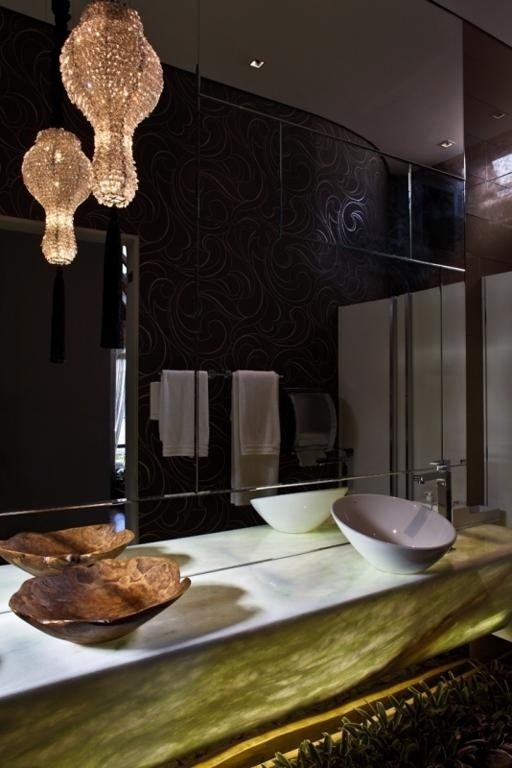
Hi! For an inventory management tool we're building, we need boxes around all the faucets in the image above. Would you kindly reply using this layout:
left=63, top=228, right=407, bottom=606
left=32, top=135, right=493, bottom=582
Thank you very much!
left=412, top=460, right=451, bottom=523
left=316, top=448, right=353, bottom=489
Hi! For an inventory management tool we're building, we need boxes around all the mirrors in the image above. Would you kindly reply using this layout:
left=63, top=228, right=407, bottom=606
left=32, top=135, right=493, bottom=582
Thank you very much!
left=0, top=0, right=467, bottom=565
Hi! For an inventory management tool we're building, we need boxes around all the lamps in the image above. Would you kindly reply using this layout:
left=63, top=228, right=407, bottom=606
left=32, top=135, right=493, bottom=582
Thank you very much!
left=59, top=0, right=165, bottom=349
left=20, top=1, right=94, bottom=363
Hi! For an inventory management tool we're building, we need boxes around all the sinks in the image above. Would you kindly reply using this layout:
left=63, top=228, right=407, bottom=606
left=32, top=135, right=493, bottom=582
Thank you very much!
left=250, top=486, right=349, bottom=533
left=332, top=493, right=455, bottom=575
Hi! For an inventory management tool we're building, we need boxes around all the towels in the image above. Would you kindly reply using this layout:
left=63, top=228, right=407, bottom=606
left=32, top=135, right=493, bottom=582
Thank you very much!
left=159, top=369, right=209, bottom=458
left=230, top=369, right=281, bottom=506
